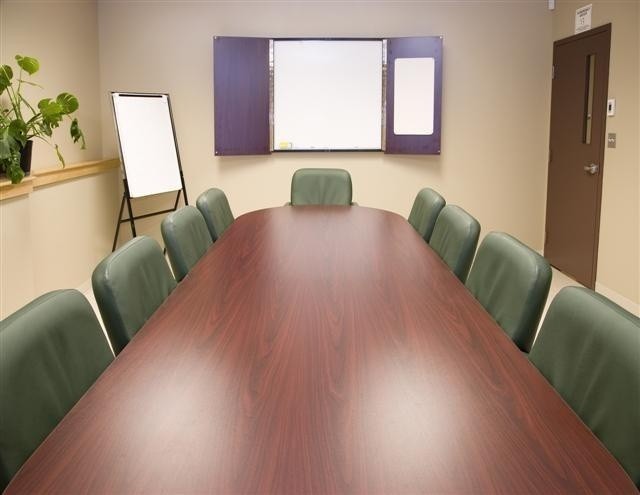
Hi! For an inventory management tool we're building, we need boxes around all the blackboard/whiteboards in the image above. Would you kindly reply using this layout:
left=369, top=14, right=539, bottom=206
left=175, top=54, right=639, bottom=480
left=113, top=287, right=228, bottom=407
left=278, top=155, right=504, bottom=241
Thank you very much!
left=109, top=91, right=184, bottom=198
left=269, top=39, right=387, bottom=150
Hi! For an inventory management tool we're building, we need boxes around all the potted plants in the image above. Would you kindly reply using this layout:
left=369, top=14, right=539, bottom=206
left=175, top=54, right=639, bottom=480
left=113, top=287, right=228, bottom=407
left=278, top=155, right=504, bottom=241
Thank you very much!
left=0, top=53, right=87, bottom=185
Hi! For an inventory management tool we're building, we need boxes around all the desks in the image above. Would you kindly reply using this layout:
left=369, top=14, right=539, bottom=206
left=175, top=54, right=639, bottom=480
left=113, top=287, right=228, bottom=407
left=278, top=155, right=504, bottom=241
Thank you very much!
left=0, top=204, right=640, bottom=495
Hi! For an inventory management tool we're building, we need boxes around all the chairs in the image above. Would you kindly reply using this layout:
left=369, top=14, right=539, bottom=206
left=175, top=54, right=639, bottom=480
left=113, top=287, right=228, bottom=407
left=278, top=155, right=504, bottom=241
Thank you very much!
left=281, top=167, right=361, bottom=208
left=528, top=283, right=640, bottom=491
left=0, top=285, right=117, bottom=494
left=159, top=205, right=215, bottom=283
left=407, top=187, right=447, bottom=243
left=462, top=228, right=554, bottom=352
left=90, top=234, right=177, bottom=357
left=195, top=186, right=237, bottom=243
left=428, top=204, right=481, bottom=286
